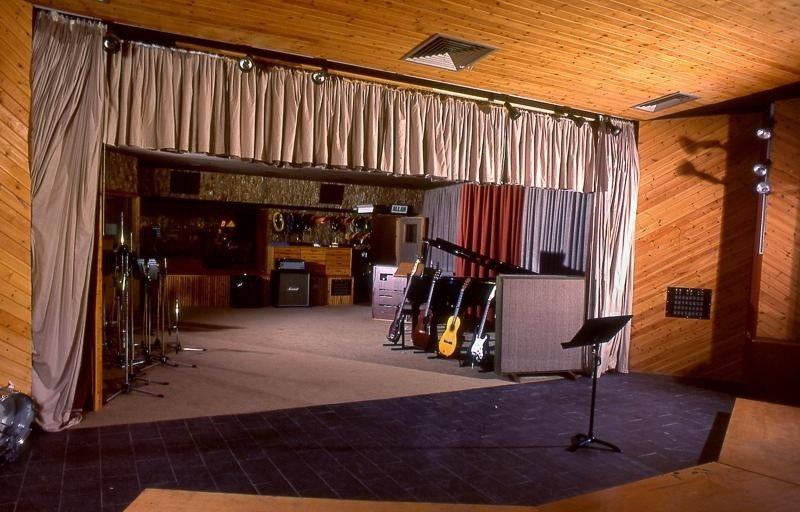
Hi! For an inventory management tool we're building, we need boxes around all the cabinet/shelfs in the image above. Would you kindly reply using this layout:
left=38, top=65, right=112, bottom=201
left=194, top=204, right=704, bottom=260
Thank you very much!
left=267, top=246, right=352, bottom=277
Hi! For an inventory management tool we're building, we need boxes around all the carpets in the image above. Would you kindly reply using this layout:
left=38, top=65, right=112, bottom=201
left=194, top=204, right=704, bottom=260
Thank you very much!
left=1, top=372, right=737, bottom=511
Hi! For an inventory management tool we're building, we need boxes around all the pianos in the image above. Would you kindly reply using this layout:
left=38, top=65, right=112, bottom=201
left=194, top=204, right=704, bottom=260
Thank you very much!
left=406, top=238, right=539, bottom=333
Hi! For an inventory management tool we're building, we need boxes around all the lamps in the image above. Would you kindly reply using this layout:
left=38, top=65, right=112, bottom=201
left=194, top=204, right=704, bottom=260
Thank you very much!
left=503, top=100, right=622, bottom=136
left=240, top=54, right=254, bottom=72
left=103, top=33, right=121, bottom=54
left=313, top=65, right=329, bottom=85
left=754, top=114, right=773, bottom=196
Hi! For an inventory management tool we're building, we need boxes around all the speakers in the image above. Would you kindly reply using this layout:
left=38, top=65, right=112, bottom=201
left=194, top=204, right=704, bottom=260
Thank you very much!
left=270, top=269, right=310, bottom=307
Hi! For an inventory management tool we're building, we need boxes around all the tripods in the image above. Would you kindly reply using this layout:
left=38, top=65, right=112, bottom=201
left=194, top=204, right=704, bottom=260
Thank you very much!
left=571, top=333, right=624, bottom=453
left=103, top=209, right=207, bottom=406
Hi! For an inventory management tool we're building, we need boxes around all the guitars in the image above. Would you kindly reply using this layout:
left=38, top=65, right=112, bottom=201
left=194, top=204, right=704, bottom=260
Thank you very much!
left=437, top=277, right=471, bottom=359
left=412, top=270, right=441, bottom=350
left=389, top=256, right=424, bottom=342
left=466, top=285, right=496, bottom=363
